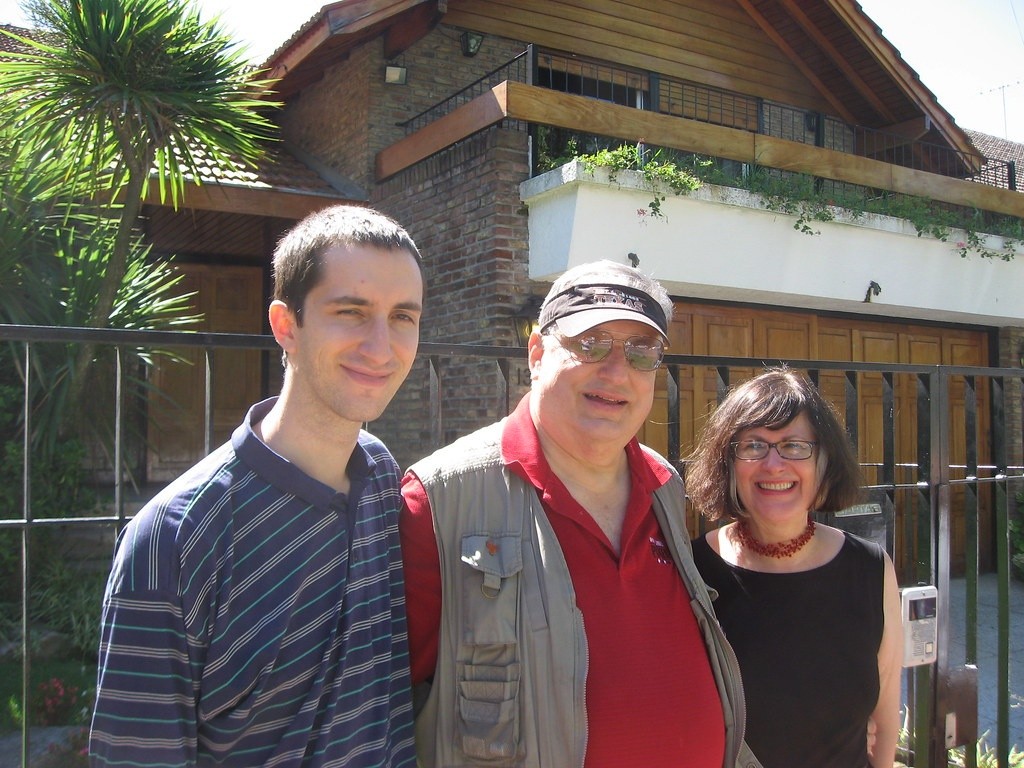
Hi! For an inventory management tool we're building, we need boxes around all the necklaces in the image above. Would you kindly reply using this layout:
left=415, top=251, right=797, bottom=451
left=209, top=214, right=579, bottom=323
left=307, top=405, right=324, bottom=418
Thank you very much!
left=736, top=517, right=816, bottom=560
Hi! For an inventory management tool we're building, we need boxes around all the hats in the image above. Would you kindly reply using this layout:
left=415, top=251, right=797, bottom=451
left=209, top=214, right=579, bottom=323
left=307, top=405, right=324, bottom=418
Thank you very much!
left=539, top=283, right=671, bottom=349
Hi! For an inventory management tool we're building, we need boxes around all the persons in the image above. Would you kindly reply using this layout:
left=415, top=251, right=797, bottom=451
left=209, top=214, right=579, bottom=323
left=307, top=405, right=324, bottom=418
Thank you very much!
left=688, top=371, right=903, bottom=768
left=88, top=207, right=425, bottom=768
left=402, top=261, right=879, bottom=768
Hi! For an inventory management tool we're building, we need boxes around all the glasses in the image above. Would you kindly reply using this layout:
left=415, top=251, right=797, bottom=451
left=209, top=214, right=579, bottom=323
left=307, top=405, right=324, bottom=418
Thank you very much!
left=731, top=439, right=820, bottom=461
left=540, top=329, right=665, bottom=372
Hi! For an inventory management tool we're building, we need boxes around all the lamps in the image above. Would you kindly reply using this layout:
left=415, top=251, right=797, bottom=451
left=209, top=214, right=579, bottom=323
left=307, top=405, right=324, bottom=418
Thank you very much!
left=806, top=109, right=818, bottom=131
left=460, top=32, right=484, bottom=56
left=384, top=53, right=407, bottom=85
